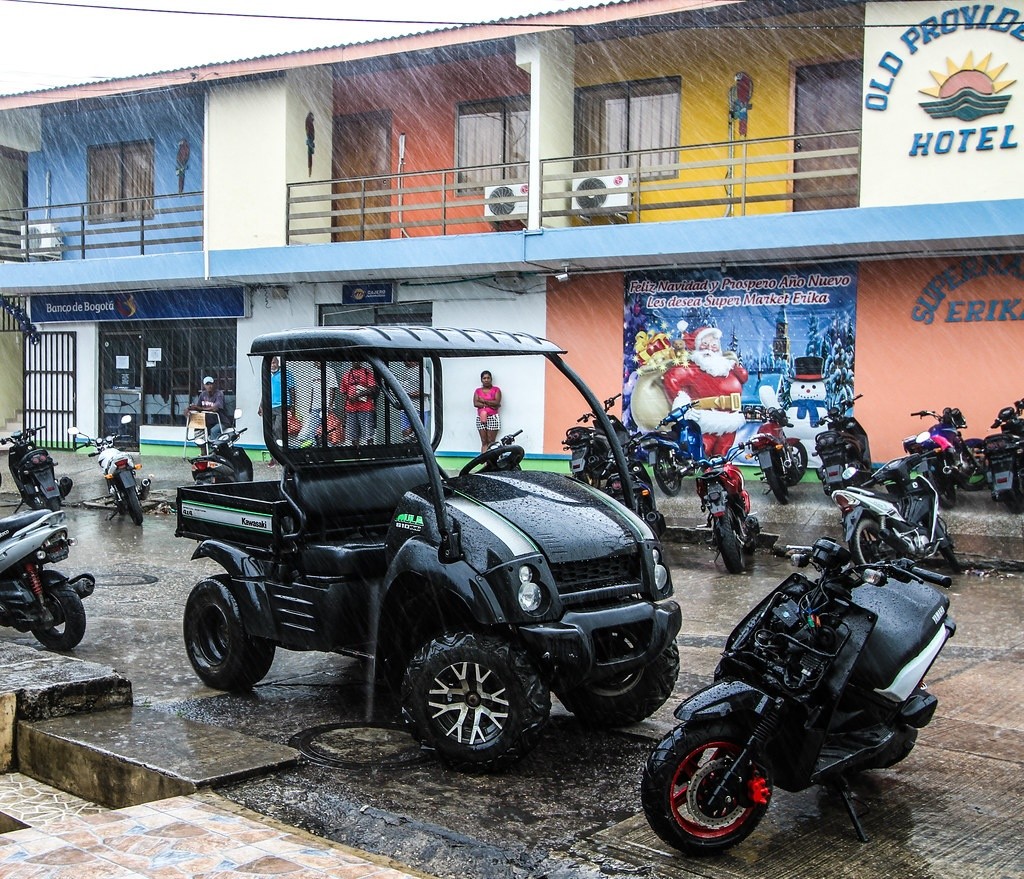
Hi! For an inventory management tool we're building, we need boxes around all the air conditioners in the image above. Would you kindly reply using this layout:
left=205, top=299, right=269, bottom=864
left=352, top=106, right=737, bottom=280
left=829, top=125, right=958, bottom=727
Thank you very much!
left=19, top=220, right=63, bottom=257
left=484, top=183, right=529, bottom=216
left=570, top=172, right=635, bottom=212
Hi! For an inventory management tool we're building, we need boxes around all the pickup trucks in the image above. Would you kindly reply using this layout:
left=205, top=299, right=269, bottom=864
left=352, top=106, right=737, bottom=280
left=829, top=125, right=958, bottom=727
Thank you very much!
left=174, top=323, right=684, bottom=774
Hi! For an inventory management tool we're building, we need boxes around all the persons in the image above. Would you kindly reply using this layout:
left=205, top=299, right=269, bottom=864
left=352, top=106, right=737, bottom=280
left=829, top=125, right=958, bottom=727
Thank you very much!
left=474, top=371, right=502, bottom=453
left=185, top=376, right=225, bottom=456
left=340, top=361, right=380, bottom=445
left=298, top=361, right=339, bottom=446
left=401, top=360, right=430, bottom=437
left=258, top=356, right=295, bottom=466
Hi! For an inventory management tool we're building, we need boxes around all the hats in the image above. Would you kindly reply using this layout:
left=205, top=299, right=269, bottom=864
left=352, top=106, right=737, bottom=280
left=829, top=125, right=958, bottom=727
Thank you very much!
left=203, top=376, right=214, bottom=385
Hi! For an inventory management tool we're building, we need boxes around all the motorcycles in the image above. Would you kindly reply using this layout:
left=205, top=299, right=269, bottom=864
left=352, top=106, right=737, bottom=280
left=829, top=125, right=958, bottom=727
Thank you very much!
left=0, top=509, right=96, bottom=652
left=188, top=408, right=254, bottom=485
left=562, top=393, right=707, bottom=535
left=67, top=415, right=152, bottom=526
left=830, top=431, right=961, bottom=576
left=0, top=423, right=73, bottom=519
left=679, top=439, right=761, bottom=575
left=905, top=398, right=1024, bottom=514
left=641, top=535, right=958, bottom=856
left=739, top=393, right=871, bottom=506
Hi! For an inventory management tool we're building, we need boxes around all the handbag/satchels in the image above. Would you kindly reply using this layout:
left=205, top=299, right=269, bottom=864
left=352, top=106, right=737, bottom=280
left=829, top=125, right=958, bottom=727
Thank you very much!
left=480, top=403, right=487, bottom=423
left=287, top=416, right=302, bottom=434
left=318, top=413, right=344, bottom=443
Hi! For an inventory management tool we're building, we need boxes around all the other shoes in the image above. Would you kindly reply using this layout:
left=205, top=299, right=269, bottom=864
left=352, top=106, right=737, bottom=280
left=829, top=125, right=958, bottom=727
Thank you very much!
left=267, top=459, right=275, bottom=468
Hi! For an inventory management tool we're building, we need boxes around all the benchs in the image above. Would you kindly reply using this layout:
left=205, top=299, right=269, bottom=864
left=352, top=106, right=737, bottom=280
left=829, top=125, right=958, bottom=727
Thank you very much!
left=294, top=458, right=429, bottom=574
